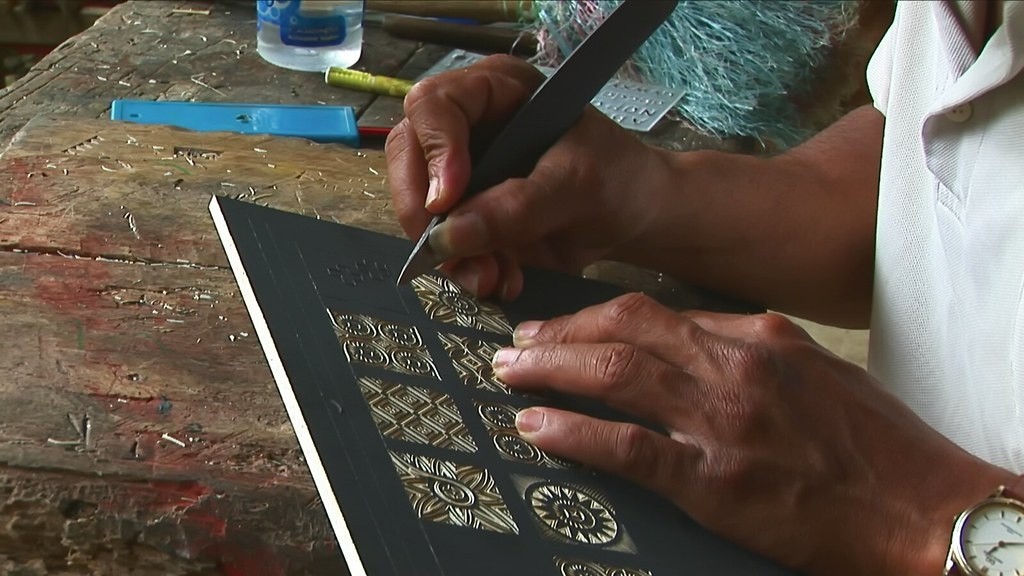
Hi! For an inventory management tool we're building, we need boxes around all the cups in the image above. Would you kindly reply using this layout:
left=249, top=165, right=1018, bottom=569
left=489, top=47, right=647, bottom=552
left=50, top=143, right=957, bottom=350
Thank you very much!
left=256, top=0, right=364, bottom=73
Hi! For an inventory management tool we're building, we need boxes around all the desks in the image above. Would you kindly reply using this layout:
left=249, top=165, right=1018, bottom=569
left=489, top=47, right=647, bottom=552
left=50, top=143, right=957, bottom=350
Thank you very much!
left=0, top=1, right=735, bottom=576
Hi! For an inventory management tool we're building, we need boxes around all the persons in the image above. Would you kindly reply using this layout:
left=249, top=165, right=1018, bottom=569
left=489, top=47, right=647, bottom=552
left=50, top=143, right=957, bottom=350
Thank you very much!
left=380, top=0, right=1023, bottom=574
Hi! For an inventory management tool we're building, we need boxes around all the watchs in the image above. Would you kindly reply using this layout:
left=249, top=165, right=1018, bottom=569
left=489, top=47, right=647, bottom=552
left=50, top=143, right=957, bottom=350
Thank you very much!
left=943, top=473, right=1024, bottom=576
